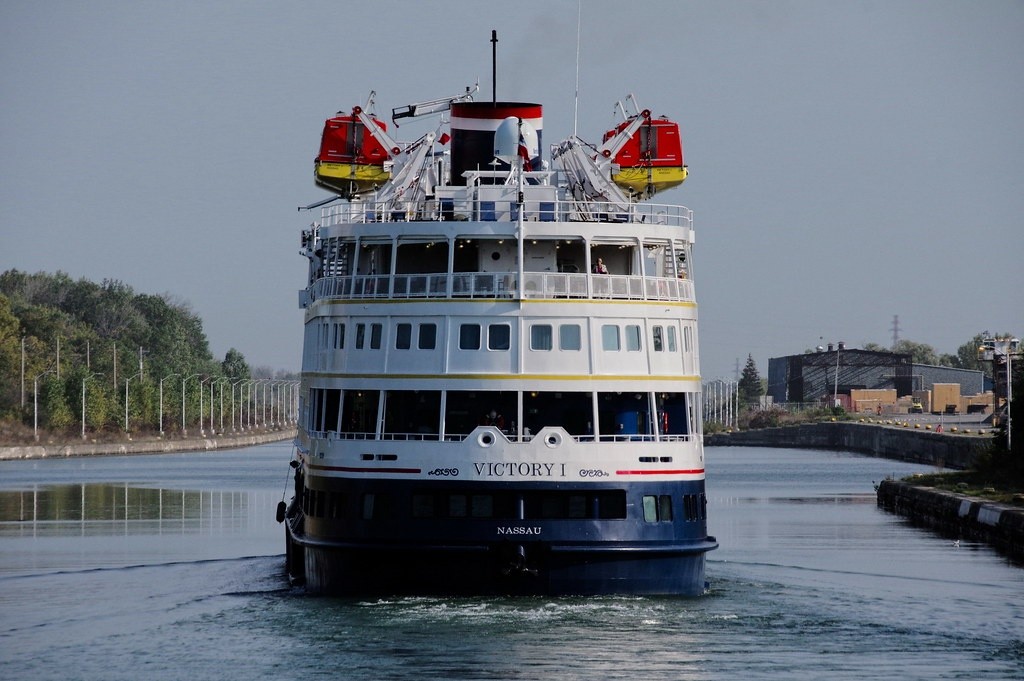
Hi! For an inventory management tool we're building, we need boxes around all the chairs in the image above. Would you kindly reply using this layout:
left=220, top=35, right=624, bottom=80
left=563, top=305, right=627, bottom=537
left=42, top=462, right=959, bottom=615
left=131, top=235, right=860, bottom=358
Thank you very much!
left=481, top=202, right=498, bottom=222
left=496, top=275, right=511, bottom=298
left=475, top=275, right=493, bottom=297
left=440, top=198, right=455, bottom=222
left=539, top=202, right=555, bottom=222
left=511, top=202, right=529, bottom=222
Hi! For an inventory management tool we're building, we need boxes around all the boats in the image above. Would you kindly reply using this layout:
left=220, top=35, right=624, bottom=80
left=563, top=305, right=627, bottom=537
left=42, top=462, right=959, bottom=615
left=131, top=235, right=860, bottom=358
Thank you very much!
left=283, top=26, right=722, bottom=601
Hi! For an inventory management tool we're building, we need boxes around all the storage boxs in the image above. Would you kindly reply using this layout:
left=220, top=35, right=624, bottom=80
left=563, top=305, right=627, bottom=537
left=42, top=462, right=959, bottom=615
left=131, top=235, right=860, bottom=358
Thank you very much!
left=392, top=212, right=407, bottom=221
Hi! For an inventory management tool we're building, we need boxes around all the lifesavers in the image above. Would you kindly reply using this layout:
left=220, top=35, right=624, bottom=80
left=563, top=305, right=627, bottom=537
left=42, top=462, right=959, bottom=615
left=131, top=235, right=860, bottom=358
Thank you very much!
left=484, top=412, right=505, bottom=430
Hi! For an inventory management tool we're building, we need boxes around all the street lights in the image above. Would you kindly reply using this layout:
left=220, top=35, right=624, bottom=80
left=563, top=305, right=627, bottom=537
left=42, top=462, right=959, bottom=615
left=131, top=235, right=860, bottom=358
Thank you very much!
left=160, top=375, right=181, bottom=433
left=125, top=371, right=141, bottom=433
left=34, top=370, right=57, bottom=440
left=182, top=373, right=203, bottom=433
left=702, top=380, right=739, bottom=431
left=82, top=371, right=104, bottom=434
left=978, top=344, right=1011, bottom=450
left=834, top=348, right=840, bottom=400
left=211, top=376, right=301, bottom=433
left=200, top=375, right=218, bottom=432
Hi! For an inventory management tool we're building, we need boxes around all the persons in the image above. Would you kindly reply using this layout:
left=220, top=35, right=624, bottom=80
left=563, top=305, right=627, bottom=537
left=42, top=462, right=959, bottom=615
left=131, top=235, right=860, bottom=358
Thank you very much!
left=591, top=258, right=608, bottom=299
left=485, top=410, right=506, bottom=430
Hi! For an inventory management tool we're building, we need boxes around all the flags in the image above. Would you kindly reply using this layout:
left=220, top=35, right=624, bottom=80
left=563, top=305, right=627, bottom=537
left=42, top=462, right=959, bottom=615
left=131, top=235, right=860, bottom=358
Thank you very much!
left=518, top=130, right=532, bottom=173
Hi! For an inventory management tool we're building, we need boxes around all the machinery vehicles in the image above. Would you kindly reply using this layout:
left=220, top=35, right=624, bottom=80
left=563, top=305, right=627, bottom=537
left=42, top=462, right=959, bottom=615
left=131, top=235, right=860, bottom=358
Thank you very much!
left=908, top=397, right=924, bottom=414
